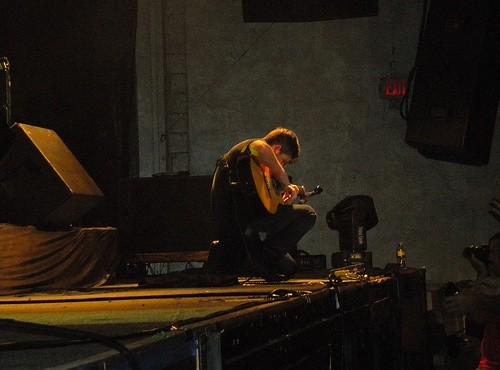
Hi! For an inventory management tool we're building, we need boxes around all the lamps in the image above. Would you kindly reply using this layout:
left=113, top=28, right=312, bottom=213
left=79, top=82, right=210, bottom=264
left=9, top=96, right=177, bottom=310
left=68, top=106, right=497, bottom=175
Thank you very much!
left=326, top=195, right=379, bottom=270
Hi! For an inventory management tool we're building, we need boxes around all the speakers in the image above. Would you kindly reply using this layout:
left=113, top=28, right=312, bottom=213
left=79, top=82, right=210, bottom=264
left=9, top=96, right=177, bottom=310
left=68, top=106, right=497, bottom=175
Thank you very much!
left=126, top=174, right=220, bottom=253
left=0, top=121, right=105, bottom=229
left=404, top=0, right=500, bottom=166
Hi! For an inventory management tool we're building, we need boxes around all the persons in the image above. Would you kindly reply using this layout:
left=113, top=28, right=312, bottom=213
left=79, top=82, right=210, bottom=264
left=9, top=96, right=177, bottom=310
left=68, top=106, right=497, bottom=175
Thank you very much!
left=210, top=126, right=317, bottom=274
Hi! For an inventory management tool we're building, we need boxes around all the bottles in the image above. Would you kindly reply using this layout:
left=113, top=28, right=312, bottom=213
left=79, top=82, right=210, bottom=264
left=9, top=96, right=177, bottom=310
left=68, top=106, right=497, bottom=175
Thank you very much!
left=397, top=242, right=406, bottom=270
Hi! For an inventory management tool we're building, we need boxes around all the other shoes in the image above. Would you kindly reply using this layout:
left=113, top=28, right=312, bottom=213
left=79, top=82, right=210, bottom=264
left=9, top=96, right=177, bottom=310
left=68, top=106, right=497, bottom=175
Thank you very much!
left=289, top=249, right=309, bottom=263
left=261, top=245, right=289, bottom=280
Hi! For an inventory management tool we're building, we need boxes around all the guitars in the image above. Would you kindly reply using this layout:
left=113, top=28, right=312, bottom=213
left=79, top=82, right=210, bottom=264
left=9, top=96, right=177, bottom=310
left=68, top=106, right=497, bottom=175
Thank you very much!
left=236, top=153, right=323, bottom=218
